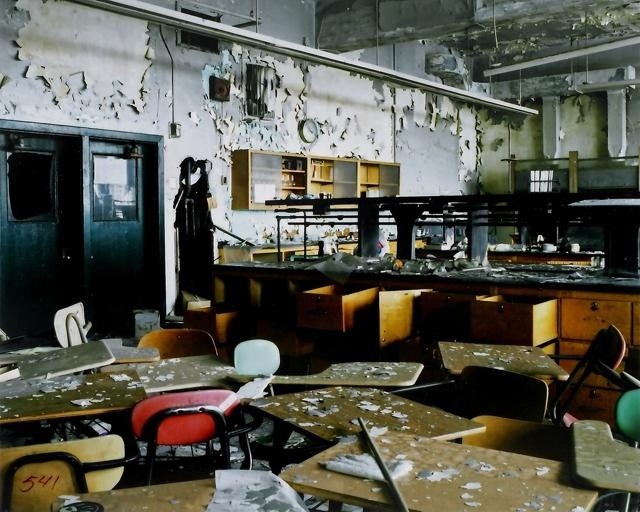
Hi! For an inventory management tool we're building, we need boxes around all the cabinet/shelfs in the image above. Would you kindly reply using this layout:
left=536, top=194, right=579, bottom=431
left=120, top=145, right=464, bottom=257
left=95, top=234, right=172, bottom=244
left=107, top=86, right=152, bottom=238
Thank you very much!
left=183, top=266, right=640, bottom=433
left=229, top=150, right=401, bottom=212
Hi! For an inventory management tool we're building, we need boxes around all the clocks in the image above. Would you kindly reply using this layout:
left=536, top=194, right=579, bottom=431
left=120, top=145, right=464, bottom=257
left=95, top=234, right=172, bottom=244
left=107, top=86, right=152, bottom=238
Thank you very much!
left=299, top=118, right=318, bottom=142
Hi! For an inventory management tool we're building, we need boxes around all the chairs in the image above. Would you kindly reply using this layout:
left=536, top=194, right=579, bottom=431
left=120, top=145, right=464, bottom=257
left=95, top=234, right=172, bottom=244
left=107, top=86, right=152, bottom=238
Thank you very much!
left=0, top=301, right=640, bottom=512
